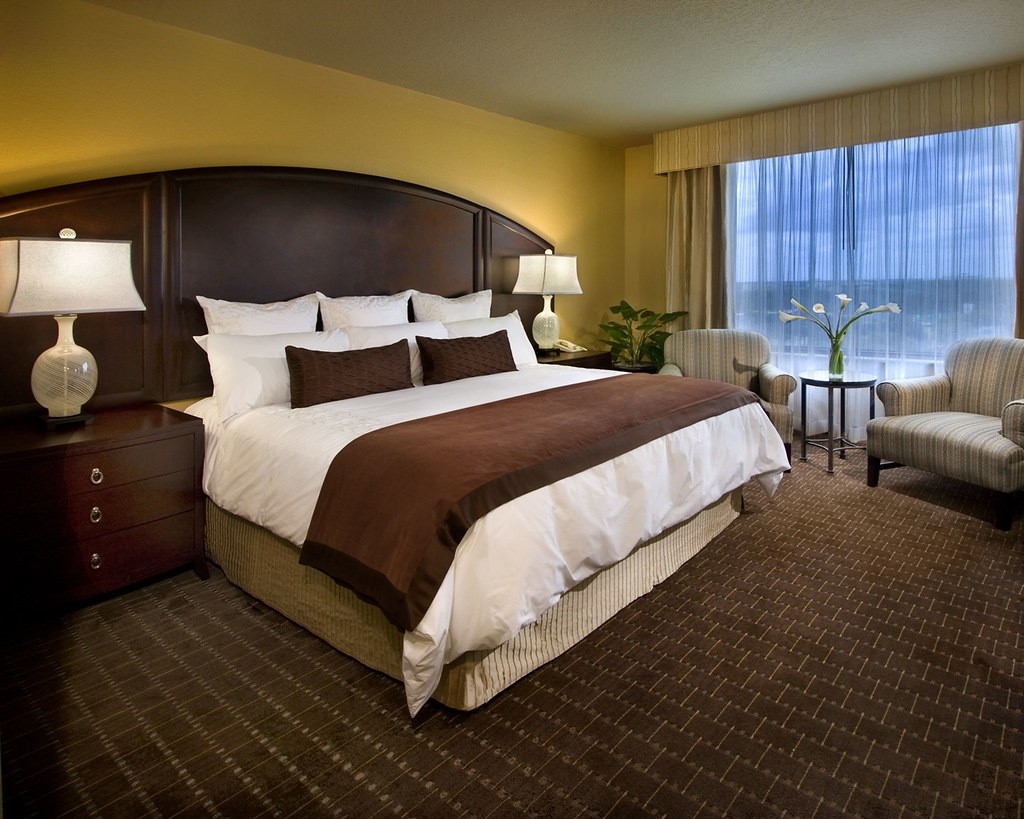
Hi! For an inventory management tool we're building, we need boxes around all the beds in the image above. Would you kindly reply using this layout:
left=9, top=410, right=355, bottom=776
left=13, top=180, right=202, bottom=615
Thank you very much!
left=184, top=363, right=792, bottom=719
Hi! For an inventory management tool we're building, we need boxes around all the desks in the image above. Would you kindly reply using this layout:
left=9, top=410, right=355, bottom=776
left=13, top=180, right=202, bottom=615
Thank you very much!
left=799, top=373, right=877, bottom=472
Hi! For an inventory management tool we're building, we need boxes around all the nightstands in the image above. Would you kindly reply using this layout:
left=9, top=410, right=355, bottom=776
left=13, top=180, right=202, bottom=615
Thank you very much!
left=537, top=349, right=610, bottom=369
left=0, top=402, right=211, bottom=625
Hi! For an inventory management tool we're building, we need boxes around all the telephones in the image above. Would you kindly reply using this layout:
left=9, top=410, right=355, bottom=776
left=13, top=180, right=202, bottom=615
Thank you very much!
left=553, top=338, right=582, bottom=353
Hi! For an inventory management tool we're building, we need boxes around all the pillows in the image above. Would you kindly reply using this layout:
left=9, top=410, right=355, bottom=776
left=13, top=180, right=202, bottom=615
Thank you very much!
left=344, top=319, right=450, bottom=389
left=192, top=329, right=350, bottom=426
left=286, top=339, right=416, bottom=409
left=409, top=289, right=492, bottom=324
left=196, top=294, right=320, bottom=333
left=415, top=330, right=519, bottom=386
left=316, top=289, right=414, bottom=331
left=445, top=309, right=538, bottom=367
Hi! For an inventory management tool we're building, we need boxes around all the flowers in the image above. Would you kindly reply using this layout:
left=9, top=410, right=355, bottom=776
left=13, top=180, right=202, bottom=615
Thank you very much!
left=778, top=294, right=902, bottom=375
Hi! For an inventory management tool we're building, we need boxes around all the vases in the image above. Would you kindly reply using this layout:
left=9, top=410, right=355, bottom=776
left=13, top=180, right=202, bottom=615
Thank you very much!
left=829, top=337, right=846, bottom=381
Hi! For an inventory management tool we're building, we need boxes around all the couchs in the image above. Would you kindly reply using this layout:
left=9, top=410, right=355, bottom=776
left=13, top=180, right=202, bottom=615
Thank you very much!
left=866, top=335, right=1024, bottom=532
left=657, top=328, right=797, bottom=473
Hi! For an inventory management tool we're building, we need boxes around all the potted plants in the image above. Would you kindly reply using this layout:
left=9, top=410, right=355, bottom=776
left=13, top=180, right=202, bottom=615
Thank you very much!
left=595, top=299, right=689, bottom=374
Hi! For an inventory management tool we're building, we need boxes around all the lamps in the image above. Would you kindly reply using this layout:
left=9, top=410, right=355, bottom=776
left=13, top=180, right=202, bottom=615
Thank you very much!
left=512, top=249, right=584, bottom=355
left=0, top=226, right=147, bottom=432
